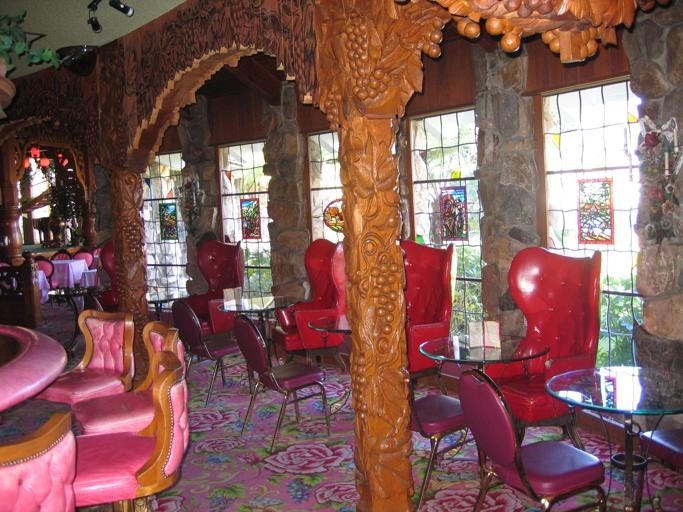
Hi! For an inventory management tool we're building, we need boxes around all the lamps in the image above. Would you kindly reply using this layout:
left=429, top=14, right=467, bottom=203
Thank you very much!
left=87, top=0, right=133, bottom=34
left=54, top=45, right=99, bottom=77
